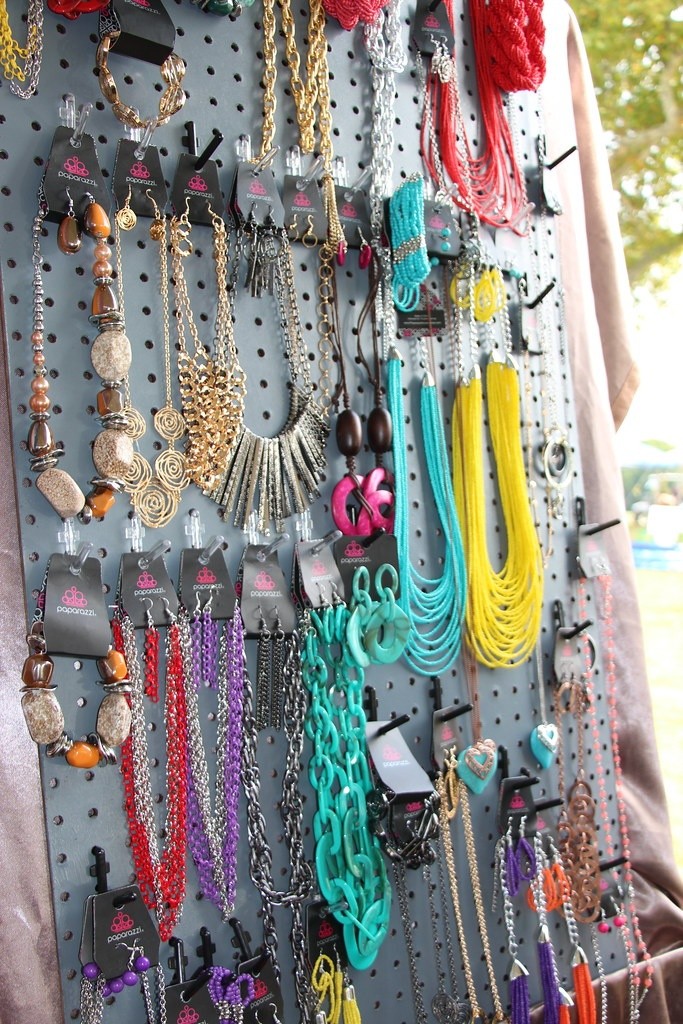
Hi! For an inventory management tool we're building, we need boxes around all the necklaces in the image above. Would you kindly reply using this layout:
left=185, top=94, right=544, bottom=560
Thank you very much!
left=281, top=0, right=320, bottom=154
left=172, top=201, right=247, bottom=489
left=0, top=0, right=44, bottom=99
left=174, top=0, right=624, bottom=675
left=262, top=0, right=276, bottom=164
left=30, top=190, right=132, bottom=523
left=71, top=520, right=650, bottom=1024
left=114, top=194, right=186, bottom=528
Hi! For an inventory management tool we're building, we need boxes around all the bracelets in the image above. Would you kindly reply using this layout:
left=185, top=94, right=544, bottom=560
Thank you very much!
left=92, top=29, right=186, bottom=130
left=21, top=622, right=130, bottom=767
